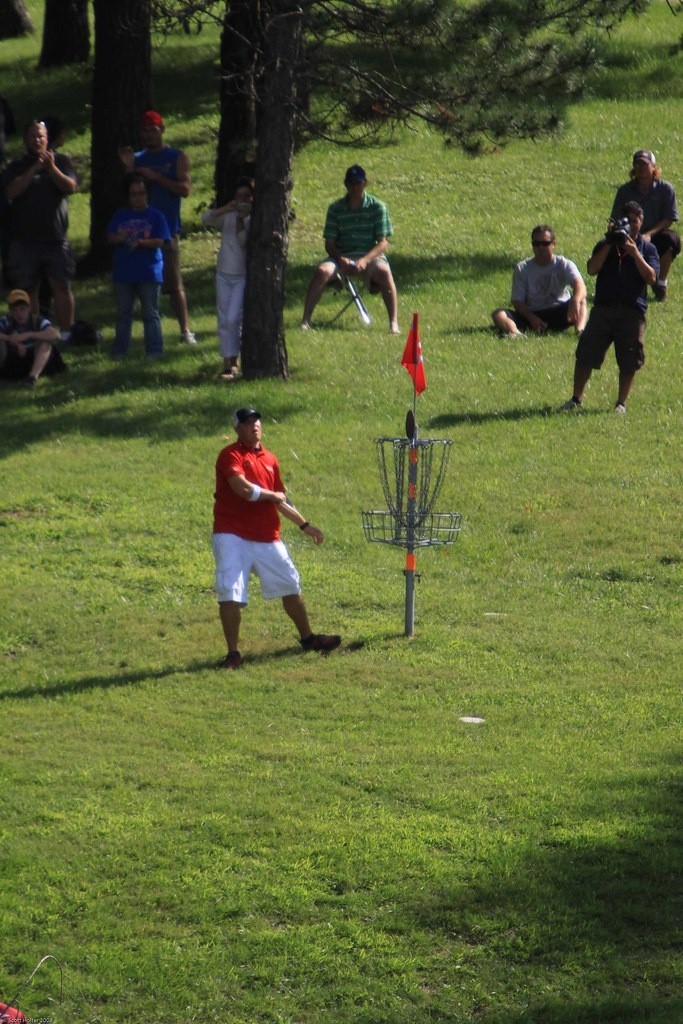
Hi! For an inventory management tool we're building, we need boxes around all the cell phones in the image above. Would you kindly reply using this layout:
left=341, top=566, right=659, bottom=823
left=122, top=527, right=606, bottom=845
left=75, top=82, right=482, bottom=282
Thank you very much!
left=605, top=218, right=631, bottom=245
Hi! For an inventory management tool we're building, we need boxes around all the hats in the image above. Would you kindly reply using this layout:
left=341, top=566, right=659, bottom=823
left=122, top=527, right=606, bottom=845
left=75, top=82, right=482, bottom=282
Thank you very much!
left=232, top=408, right=264, bottom=432
left=632, top=149, right=657, bottom=165
left=346, top=164, right=368, bottom=183
left=6, top=289, right=30, bottom=306
left=140, top=110, right=163, bottom=126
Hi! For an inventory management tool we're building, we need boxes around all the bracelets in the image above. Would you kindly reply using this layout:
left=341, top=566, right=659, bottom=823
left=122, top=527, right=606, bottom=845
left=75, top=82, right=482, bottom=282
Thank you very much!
left=248, top=483, right=260, bottom=502
left=299, top=522, right=309, bottom=530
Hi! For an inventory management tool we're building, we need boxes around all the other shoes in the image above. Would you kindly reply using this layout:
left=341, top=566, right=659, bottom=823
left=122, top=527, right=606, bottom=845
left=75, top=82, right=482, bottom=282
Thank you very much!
left=612, top=403, right=626, bottom=414
left=220, top=650, right=243, bottom=670
left=389, top=326, right=404, bottom=336
left=219, top=369, right=242, bottom=380
left=298, top=322, right=314, bottom=331
left=554, top=400, right=586, bottom=415
left=20, top=374, right=40, bottom=392
left=300, top=633, right=343, bottom=654
left=180, top=329, right=199, bottom=344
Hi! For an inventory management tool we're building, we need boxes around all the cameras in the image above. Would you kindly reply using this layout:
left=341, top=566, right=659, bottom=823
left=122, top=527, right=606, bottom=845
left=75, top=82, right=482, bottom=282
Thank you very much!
left=235, top=203, right=252, bottom=210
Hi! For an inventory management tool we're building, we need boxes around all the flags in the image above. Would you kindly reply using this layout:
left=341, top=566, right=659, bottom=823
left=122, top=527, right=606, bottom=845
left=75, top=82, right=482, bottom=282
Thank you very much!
left=401, top=313, right=426, bottom=395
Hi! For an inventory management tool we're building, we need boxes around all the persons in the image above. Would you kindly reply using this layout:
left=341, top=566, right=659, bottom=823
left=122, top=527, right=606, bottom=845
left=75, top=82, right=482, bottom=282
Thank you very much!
left=491, top=225, right=587, bottom=335
left=608, top=150, right=680, bottom=302
left=118, top=111, right=196, bottom=345
left=0, top=290, right=60, bottom=390
left=201, top=180, right=254, bottom=384
left=210, top=408, right=341, bottom=669
left=561, top=200, right=659, bottom=414
left=299, top=164, right=400, bottom=338
left=107, top=177, right=170, bottom=362
left=0, top=120, right=78, bottom=342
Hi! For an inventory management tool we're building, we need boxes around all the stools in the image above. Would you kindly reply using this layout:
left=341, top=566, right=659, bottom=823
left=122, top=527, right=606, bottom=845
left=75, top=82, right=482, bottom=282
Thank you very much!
left=326, top=274, right=372, bottom=326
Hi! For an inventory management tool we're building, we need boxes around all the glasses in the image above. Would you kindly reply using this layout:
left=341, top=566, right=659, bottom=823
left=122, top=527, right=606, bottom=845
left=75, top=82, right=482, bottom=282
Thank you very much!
left=531, top=240, right=551, bottom=247
